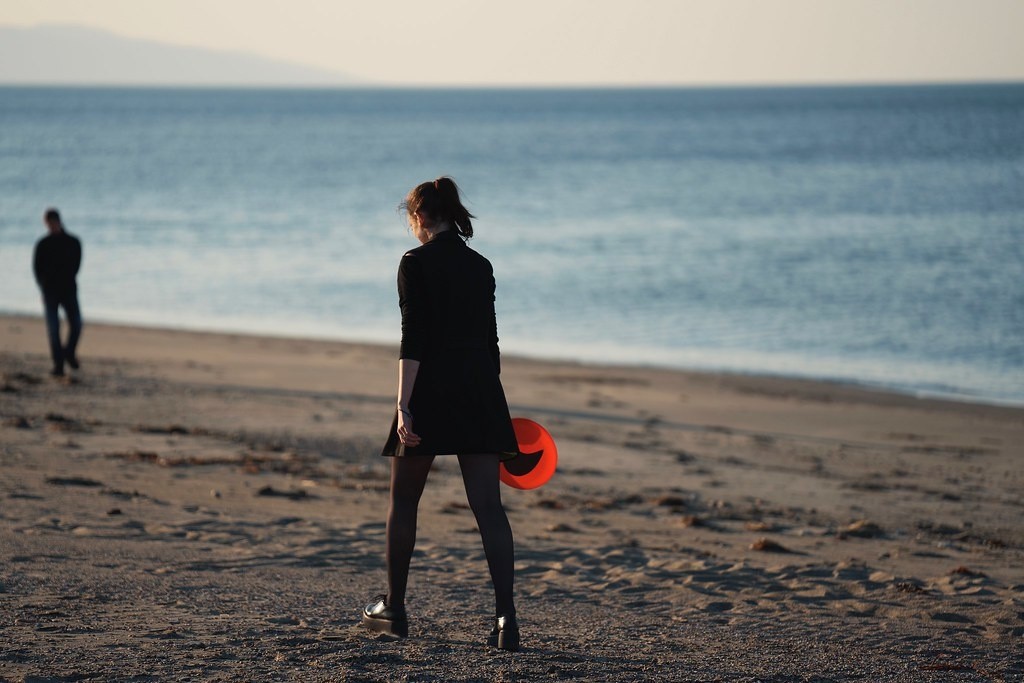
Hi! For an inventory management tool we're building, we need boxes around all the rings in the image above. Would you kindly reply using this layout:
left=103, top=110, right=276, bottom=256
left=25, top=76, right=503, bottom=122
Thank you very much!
left=400, top=435, right=405, bottom=438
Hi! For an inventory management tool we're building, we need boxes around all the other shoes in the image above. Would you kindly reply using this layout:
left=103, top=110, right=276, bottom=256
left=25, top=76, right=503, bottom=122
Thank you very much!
left=63, top=348, right=80, bottom=369
left=48, top=368, right=64, bottom=377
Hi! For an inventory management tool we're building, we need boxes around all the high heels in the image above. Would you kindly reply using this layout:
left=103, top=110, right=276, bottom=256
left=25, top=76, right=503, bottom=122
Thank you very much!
left=363, top=594, right=409, bottom=639
left=487, top=616, right=520, bottom=650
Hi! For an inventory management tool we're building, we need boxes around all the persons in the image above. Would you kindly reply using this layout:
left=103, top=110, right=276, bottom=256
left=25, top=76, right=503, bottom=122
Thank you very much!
left=33, top=211, right=82, bottom=377
left=363, top=176, right=522, bottom=651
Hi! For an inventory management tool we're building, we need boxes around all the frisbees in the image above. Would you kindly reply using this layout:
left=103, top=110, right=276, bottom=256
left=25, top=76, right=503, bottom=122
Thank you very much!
left=500, top=418, right=557, bottom=490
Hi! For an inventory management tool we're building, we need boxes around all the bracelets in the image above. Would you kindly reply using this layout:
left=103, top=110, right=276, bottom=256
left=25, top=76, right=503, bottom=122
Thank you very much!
left=396, top=408, right=414, bottom=421
